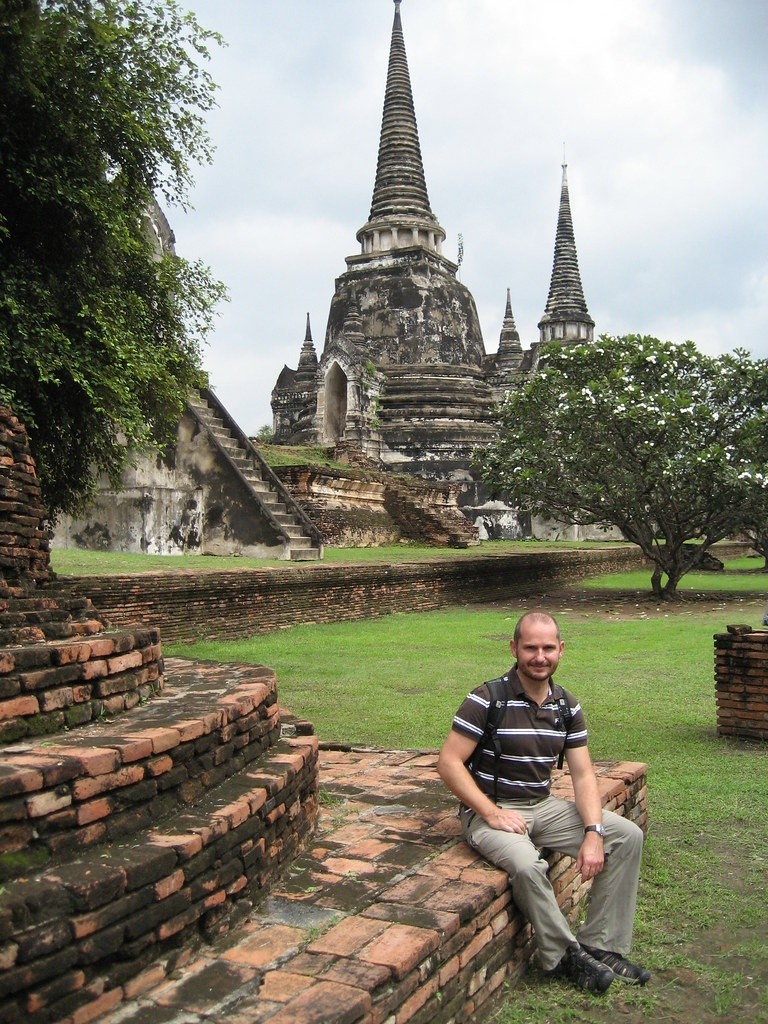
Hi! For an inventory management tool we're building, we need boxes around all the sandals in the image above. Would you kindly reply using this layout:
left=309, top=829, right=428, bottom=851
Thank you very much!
left=581, top=942, right=651, bottom=984
left=563, top=946, right=614, bottom=994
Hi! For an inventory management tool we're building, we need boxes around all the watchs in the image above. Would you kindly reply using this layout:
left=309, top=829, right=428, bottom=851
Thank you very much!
left=585, top=824, right=606, bottom=838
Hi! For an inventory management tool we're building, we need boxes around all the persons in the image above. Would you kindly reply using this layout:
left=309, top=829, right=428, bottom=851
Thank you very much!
left=437, top=612, right=651, bottom=996
